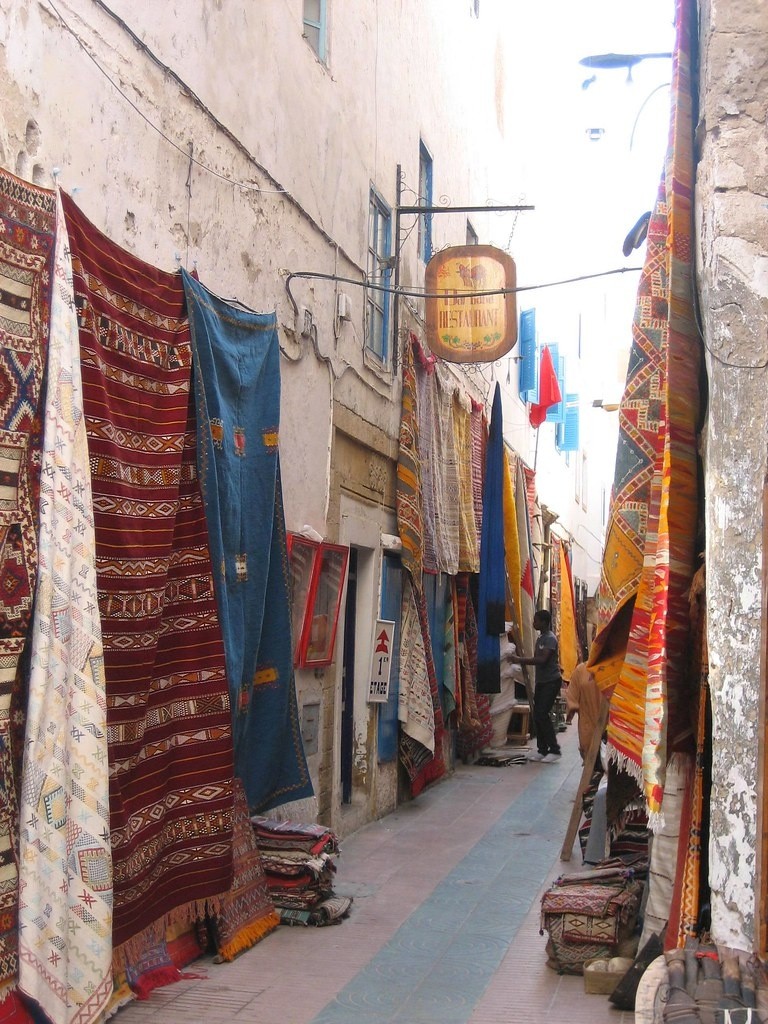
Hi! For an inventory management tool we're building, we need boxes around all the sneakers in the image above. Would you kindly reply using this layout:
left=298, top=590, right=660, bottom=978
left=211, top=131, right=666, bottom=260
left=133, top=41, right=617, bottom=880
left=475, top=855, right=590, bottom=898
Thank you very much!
left=528, top=753, right=545, bottom=761
left=540, top=752, right=561, bottom=762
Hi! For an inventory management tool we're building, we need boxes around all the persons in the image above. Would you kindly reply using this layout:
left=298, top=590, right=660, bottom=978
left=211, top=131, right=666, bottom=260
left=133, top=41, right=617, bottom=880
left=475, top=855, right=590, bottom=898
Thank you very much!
left=506, top=610, right=562, bottom=763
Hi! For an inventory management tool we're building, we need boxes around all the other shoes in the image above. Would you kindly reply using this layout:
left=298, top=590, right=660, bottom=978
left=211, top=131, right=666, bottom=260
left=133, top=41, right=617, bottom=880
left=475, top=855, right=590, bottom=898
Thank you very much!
left=564, top=721, right=572, bottom=725
